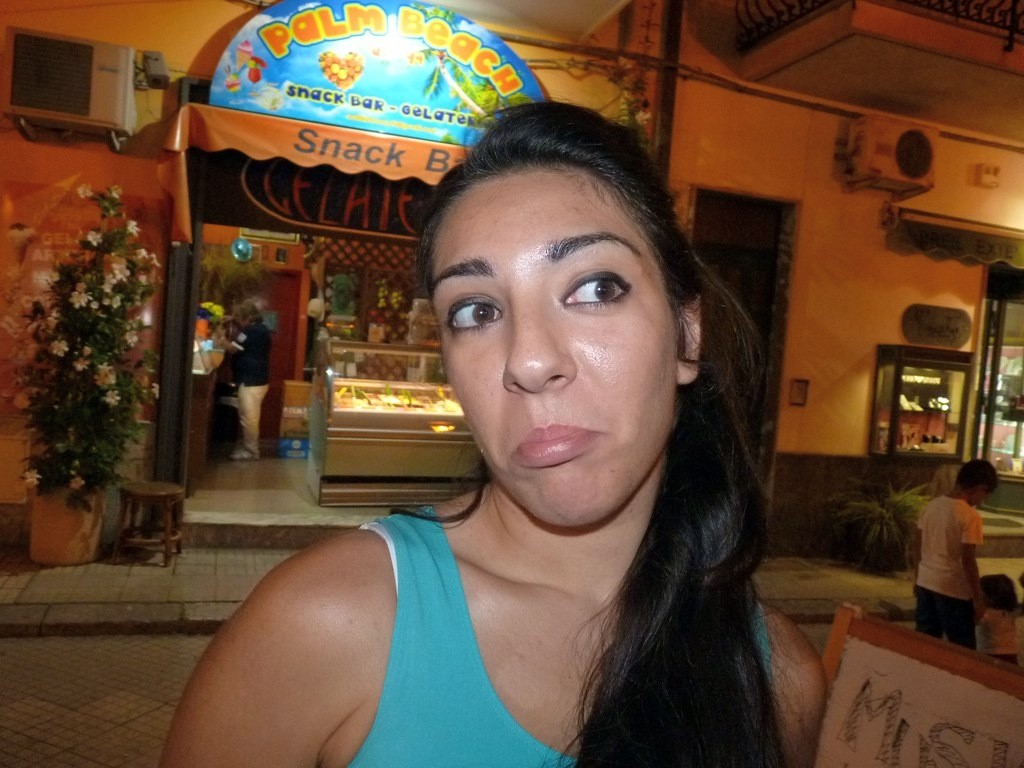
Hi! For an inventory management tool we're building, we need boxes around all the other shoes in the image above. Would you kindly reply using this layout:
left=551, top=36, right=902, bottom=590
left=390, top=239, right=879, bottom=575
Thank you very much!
left=229, top=448, right=260, bottom=460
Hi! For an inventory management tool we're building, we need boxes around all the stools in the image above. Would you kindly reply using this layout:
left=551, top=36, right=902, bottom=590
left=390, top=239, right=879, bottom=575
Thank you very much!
left=113, top=480, right=186, bottom=569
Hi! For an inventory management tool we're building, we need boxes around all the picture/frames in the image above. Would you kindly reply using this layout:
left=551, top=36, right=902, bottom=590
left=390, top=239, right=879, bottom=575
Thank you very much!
left=250, top=243, right=262, bottom=263
left=239, top=226, right=300, bottom=245
left=274, top=247, right=289, bottom=264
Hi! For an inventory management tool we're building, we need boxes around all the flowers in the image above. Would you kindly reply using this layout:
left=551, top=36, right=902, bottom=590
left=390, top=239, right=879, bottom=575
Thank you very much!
left=7, top=184, right=162, bottom=512
left=369, top=277, right=402, bottom=327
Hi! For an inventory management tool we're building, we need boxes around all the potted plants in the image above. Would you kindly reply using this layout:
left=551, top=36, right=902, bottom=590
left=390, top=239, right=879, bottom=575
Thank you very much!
left=325, top=274, right=356, bottom=340
left=839, top=481, right=936, bottom=572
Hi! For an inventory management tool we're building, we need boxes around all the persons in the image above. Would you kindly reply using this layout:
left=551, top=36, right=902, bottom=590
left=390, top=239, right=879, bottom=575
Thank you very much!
left=156, top=98, right=829, bottom=768
left=912, top=457, right=1022, bottom=667
left=217, top=298, right=273, bottom=461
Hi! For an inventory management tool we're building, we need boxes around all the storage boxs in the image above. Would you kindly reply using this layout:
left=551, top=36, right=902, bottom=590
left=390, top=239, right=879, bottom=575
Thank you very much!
left=280, top=416, right=311, bottom=439
left=280, top=439, right=309, bottom=459
left=280, top=379, right=312, bottom=418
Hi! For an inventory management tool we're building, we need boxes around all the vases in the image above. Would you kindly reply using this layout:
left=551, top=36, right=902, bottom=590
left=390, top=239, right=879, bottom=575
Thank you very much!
left=28, top=484, right=107, bottom=566
left=369, top=323, right=388, bottom=343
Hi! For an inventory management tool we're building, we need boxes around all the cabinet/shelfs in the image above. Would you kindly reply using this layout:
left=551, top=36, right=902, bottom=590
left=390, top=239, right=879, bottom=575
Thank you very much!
left=308, top=338, right=483, bottom=508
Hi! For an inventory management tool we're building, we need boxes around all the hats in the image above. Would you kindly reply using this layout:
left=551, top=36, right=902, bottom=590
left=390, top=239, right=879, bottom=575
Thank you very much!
left=307, top=289, right=324, bottom=322
left=232, top=299, right=262, bottom=321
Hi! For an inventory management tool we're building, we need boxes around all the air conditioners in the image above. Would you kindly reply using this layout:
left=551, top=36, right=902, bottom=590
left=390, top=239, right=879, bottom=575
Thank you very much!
left=847, top=113, right=939, bottom=192
